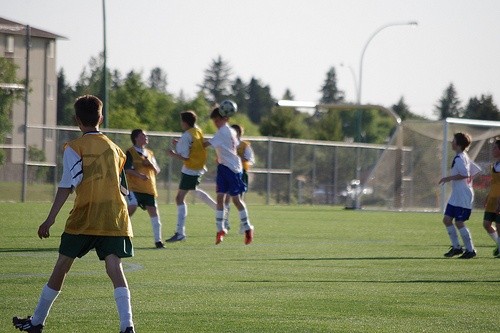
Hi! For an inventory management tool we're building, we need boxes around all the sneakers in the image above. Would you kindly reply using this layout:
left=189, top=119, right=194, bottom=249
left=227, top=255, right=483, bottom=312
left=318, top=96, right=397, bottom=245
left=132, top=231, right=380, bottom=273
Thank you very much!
left=120, top=326, right=135, bottom=333
left=493, top=248, right=500, bottom=257
left=459, top=250, right=476, bottom=258
left=12, top=315, right=45, bottom=333
left=444, top=245, right=463, bottom=257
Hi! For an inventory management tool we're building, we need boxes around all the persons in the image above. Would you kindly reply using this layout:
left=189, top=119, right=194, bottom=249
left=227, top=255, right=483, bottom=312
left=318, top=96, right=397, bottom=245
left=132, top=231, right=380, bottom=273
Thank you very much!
left=165, top=111, right=216, bottom=242
left=124, top=129, right=166, bottom=248
left=225, top=124, right=255, bottom=230
left=204, top=108, right=254, bottom=245
left=13, top=95, right=135, bottom=333
left=484, top=139, right=500, bottom=258
left=439, top=132, right=482, bottom=259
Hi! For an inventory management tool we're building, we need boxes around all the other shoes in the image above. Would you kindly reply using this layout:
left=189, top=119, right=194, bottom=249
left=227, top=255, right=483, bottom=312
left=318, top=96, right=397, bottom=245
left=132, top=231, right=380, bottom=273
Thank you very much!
left=216, top=229, right=226, bottom=245
left=244, top=228, right=253, bottom=244
left=166, top=232, right=185, bottom=241
left=155, top=241, right=166, bottom=248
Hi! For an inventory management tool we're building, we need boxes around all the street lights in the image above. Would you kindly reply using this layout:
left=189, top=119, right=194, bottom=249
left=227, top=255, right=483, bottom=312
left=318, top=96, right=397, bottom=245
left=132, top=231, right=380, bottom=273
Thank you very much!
left=355, top=20, right=419, bottom=179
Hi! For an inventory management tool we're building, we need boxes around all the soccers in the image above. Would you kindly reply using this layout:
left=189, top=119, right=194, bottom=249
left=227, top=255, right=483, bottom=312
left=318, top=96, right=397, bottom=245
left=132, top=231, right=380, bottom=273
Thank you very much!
left=218, top=99, right=239, bottom=119
